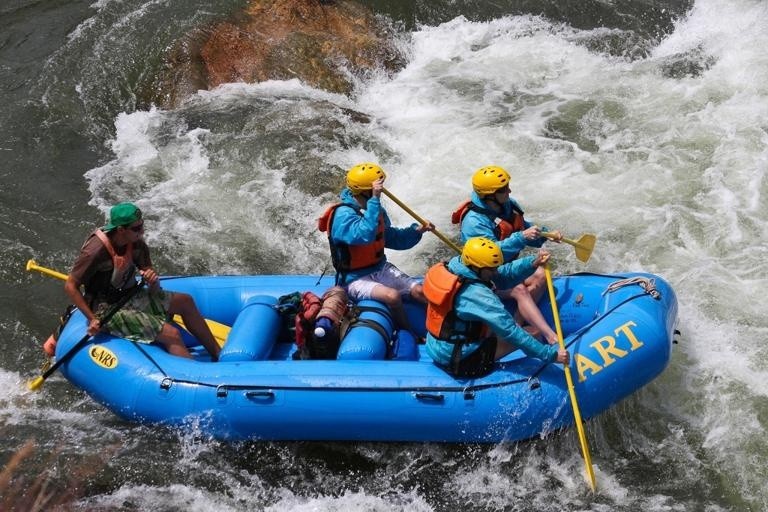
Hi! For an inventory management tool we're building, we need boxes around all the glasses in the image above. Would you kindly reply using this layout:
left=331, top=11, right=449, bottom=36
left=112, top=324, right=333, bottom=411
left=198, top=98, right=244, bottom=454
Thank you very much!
left=125, top=218, right=144, bottom=233
left=495, top=183, right=509, bottom=193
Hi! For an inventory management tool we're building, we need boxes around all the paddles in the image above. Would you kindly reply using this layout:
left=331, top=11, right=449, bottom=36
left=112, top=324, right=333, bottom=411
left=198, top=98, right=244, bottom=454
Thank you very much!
left=25, top=259, right=231, bottom=350
left=542, top=232, right=596, bottom=263
left=542, top=256, right=597, bottom=493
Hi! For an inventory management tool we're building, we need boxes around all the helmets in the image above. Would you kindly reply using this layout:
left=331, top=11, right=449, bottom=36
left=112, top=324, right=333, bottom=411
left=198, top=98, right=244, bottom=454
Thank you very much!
left=462, top=237, right=504, bottom=268
left=344, top=162, right=386, bottom=196
left=472, top=165, right=511, bottom=194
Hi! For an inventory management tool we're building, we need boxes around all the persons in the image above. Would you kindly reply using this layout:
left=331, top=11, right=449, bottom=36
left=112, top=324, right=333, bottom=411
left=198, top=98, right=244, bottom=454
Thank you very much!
left=326, top=164, right=437, bottom=346
left=63, top=202, right=222, bottom=362
left=459, top=166, right=563, bottom=345
left=426, top=237, right=571, bottom=377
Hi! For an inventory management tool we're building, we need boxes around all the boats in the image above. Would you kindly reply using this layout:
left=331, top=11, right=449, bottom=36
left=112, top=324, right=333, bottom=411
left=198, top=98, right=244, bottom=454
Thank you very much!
left=55, top=271, right=676, bottom=446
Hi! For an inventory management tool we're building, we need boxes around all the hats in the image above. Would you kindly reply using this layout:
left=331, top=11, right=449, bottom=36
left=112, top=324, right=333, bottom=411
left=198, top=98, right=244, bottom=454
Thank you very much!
left=98, top=202, right=141, bottom=231
